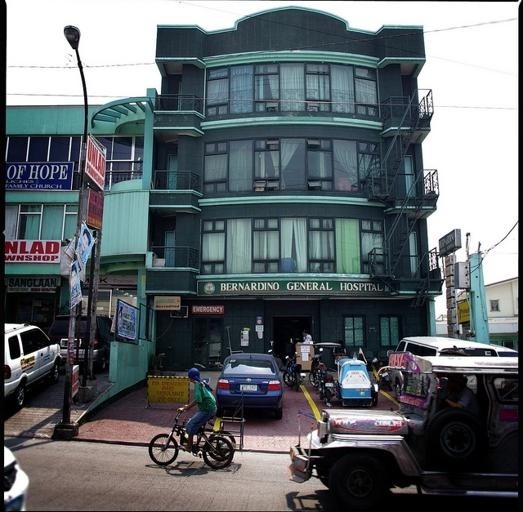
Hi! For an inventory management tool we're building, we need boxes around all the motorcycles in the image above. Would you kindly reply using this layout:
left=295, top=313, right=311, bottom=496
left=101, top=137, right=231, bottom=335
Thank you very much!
left=284, top=353, right=302, bottom=391
left=307, top=349, right=327, bottom=387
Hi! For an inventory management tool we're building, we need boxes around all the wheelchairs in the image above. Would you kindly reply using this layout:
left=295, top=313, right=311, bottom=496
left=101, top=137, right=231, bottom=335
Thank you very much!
left=316, top=375, right=341, bottom=407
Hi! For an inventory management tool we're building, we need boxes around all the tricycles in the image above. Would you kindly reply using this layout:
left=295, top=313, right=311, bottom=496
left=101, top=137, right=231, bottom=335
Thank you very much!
left=149, top=407, right=238, bottom=470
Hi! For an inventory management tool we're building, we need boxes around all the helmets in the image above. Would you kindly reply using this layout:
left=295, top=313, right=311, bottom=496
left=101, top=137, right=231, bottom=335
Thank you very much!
left=188, top=368, right=200, bottom=378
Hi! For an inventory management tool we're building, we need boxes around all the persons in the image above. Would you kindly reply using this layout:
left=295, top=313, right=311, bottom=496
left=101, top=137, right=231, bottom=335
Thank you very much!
left=71, top=264, right=79, bottom=294
left=446, top=376, right=474, bottom=409
left=177, top=368, right=218, bottom=452
left=302, top=329, right=312, bottom=343
left=80, top=228, right=91, bottom=258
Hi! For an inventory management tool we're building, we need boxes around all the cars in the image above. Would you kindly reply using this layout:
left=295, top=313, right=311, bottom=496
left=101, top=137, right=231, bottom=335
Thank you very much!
left=338, top=358, right=379, bottom=407
left=215, top=352, right=283, bottom=419
left=290, top=352, right=522, bottom=512
left=4, top=322, right=63, bottom=406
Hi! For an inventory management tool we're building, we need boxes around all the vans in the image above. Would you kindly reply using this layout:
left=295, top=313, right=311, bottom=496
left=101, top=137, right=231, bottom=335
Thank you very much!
left=387, top=336, right=499, bottom=358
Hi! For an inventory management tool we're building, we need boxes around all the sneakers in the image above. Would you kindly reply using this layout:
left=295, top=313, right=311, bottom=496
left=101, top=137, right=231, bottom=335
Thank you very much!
left=180, top=445, right=192, bottom=452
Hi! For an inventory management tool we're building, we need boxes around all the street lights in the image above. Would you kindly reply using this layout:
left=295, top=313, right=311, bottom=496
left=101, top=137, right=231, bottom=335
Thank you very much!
left=54, top=26, right=89, bottom=437
